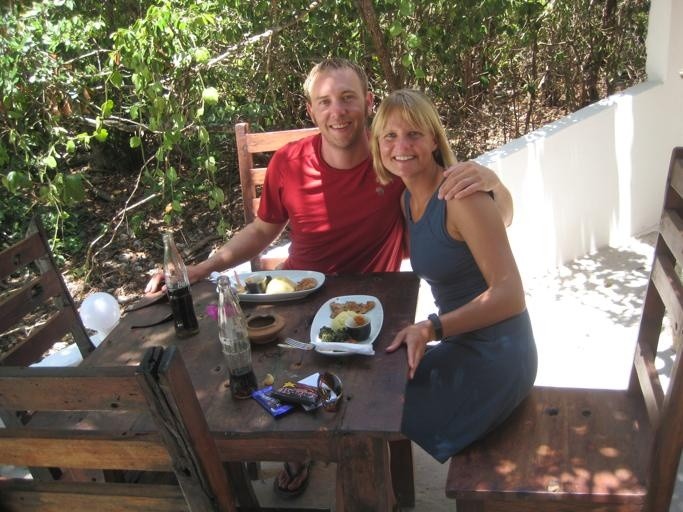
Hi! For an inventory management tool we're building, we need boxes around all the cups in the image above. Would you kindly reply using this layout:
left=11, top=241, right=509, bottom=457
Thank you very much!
left=317, top=372, right=344, bottom=411
left=123, top=292, right=175, bottom=329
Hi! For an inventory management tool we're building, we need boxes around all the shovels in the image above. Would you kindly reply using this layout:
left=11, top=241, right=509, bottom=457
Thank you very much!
left=295, top=278, right=318, bottom=291
left=329, top=301, right=375, bottom=319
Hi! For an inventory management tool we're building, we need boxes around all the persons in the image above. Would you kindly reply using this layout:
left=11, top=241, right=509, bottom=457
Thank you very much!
left=142, top=58, right=514, bottom=500
left=369, top=89, right=537, bottom=512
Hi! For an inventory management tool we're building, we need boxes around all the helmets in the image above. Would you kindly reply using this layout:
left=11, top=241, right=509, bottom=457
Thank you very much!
left=216, top=270, right=326, bottom=304
left=311, top=294, right=384, bottom=356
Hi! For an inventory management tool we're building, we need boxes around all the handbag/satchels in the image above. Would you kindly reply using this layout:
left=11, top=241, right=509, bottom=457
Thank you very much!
left=428, top=313, right=443, bottom=341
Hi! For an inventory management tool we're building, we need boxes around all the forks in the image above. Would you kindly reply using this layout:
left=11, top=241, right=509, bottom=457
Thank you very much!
left=285, top=337, right=315, bottom=351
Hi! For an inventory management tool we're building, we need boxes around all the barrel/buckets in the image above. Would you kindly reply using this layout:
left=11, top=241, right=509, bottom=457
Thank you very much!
left=274, top=462, right=311, bottom=497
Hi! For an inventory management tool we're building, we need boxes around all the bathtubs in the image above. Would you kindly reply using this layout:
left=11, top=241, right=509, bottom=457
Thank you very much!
left=318, top=325, right=348, bottom=342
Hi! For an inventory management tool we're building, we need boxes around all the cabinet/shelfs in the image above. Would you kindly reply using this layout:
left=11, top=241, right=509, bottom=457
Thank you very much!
left=217, top=275, right=258, bottom=401
left=160, top=230, right=200, bottom=338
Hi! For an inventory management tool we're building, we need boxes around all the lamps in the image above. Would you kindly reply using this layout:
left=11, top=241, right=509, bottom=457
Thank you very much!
left=446, top=148, right=683, bottom=512
left=234, top=123, right=321, bottom=272
left=0, top=214, right=96, bottom=481
left=0, top=345, right=237, bottom=512
